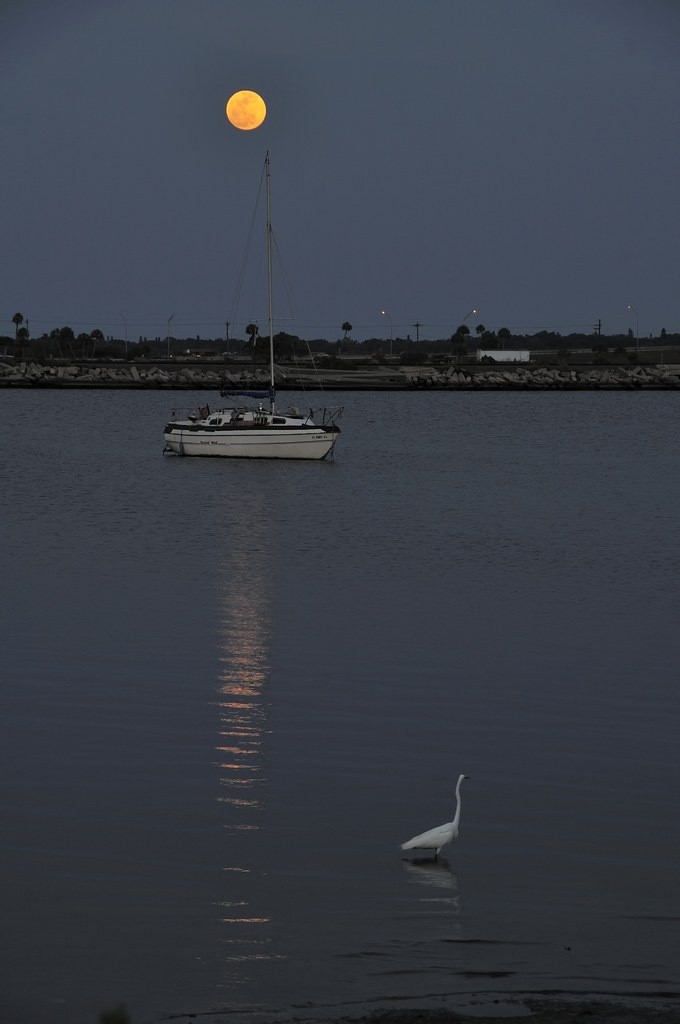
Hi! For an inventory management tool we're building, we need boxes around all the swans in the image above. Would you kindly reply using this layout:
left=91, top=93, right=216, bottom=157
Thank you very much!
left=398, top=773, right=470, bottom=860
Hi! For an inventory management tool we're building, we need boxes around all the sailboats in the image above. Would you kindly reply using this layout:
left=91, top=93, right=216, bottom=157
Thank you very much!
left=163, top=147, right=342, bottom=458
